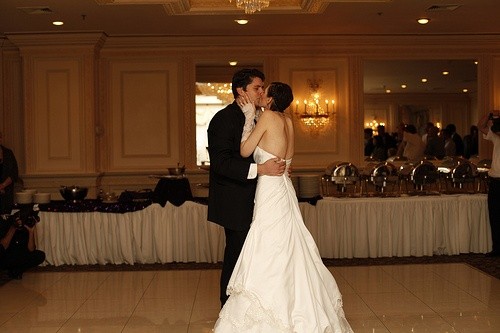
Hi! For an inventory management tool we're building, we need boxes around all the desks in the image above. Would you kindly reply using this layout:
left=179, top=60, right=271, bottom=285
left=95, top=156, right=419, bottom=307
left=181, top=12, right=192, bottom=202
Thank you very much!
left=34, top=194, right=493, bottom=267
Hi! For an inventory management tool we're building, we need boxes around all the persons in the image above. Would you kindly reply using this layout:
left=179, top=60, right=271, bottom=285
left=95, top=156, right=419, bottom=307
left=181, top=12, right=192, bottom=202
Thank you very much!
left=0, top=144, right=46, bottom=281
left=206, top=67, right=295, bottom=309
left=212, top=82, right=356, bottom=333
left=483, top=109, right=500, bottom=257
left=364, top=122, right=480, bottom=161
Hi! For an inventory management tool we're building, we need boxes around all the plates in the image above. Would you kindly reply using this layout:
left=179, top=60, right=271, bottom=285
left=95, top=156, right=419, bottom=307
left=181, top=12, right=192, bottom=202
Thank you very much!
left=22, top=189, right=38, bottom=202
left=16, top=193, right=32, bottom=204
left=299, top=175, right=320, bottom=198
left=34, top=193, right=51, bottom=203
left=290, top=175, right=299, bottom=199
left=102, top=201, right=118, bottom=203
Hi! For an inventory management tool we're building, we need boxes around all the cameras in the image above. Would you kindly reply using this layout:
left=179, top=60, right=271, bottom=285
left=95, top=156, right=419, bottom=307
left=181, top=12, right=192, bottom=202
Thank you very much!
left=7, top=204, right=41, bottom=229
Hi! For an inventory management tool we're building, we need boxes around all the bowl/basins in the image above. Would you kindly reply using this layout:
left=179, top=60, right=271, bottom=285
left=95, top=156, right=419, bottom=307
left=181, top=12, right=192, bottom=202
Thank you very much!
left=60, top=188, right=88, bottom=203
left=167, top=168, right=186, bottom=175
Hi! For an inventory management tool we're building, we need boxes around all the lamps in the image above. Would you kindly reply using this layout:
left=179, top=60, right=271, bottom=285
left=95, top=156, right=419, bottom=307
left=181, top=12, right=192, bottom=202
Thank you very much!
left=236, top=0, right=271, bottom=14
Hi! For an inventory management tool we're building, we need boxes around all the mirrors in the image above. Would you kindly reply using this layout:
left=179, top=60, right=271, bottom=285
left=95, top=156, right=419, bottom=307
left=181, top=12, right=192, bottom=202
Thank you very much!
left=361, top=60, right=479, bottom=159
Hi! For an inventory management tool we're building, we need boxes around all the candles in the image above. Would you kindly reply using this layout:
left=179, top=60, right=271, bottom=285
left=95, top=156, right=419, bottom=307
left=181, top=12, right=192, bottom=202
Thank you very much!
left=303, top=99, right=307, bottom=112
left=331, top=100, right=334, bottom=112
left=325, top=99, right=328, bottom=112
left=295, top=99, right=299, bottom=112
left=315, top=100, right=319, bottom=112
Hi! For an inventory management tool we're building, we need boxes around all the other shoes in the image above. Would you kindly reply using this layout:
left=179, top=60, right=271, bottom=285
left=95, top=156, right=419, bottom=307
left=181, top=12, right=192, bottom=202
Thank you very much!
left=485, top=250, right=500, bottom=257
